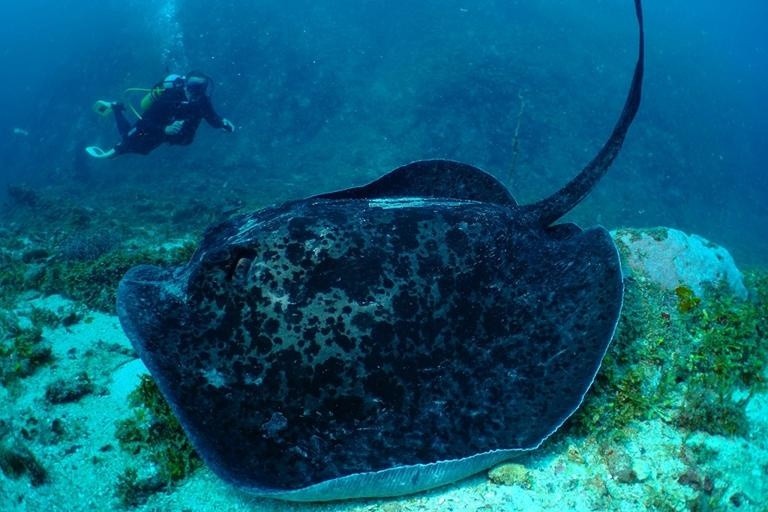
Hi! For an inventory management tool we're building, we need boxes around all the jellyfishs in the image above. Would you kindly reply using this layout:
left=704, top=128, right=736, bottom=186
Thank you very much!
left=114, top=0, right=644, bottom=503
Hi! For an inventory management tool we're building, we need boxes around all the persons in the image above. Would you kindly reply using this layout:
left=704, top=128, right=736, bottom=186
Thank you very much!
left=112, top=70, right=235, bottom=155
